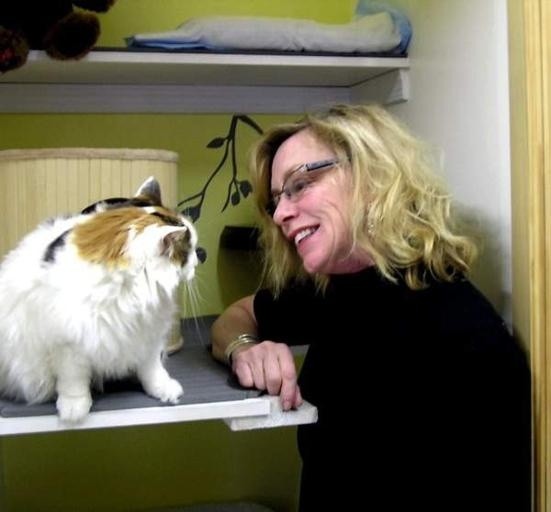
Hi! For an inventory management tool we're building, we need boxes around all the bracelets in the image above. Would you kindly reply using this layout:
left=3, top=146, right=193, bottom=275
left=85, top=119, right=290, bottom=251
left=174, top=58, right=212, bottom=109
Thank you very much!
left=223, top=333, right=259, bottom=368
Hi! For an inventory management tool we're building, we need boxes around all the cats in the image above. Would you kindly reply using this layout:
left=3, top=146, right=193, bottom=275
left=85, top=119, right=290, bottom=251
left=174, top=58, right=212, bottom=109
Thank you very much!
left=0, top=174, right=200, bottom=424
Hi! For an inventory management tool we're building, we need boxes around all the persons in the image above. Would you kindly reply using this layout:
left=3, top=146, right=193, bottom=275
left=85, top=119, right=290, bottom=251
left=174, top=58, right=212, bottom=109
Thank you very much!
left=208, top=105, right=532, bottom=512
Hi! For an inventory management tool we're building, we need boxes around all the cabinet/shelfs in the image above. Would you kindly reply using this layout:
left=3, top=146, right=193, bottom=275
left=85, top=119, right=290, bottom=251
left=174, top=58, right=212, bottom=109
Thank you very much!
left=0, top=47, right=411, bottom=438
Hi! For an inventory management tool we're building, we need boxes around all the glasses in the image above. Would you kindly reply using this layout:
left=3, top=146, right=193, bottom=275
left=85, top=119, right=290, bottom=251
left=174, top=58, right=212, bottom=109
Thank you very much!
left=271, top=154, right=353, bottom=208
